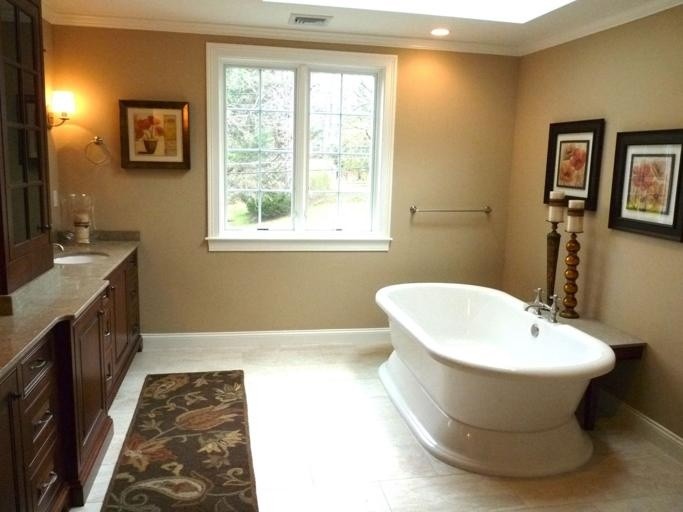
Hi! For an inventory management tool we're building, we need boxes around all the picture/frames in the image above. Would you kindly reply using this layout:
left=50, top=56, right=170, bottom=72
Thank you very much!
left=119, top=99, right=192, bottom=169
left=544, top=119, right=605, bottom=212
left=608, top=128, right=683, bottom=242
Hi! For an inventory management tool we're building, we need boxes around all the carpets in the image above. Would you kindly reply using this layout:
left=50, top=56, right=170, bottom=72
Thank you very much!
left=101, top=370, right=259, bottom=512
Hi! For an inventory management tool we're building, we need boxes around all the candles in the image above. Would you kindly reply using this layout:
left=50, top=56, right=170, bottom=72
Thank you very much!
left=549, top=191, right=564, bottom=222
left=567, top=200, right=585, bottom=232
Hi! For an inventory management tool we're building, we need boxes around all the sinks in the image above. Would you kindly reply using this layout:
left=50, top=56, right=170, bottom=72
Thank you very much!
left=53, top=252, right=109, bottom=263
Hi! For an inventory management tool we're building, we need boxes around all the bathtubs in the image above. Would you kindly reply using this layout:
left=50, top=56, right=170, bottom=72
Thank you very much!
left=376, top=283, right=616, bottom=480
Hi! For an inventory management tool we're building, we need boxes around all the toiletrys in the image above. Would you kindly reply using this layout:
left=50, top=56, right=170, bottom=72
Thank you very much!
left=73, top=207, right=90, bottom=243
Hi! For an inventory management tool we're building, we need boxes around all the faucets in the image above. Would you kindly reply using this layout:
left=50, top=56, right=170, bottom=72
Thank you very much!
left=524, top=304, right=545, bottom=312
left=52, top=242, right=65, bottom=251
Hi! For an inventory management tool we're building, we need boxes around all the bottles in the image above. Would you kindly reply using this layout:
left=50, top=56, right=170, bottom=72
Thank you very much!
left=70, top=193, right=92, bottom=247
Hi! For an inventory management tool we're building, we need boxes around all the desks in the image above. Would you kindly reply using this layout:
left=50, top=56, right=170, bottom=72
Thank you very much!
left=526, top=301, right=647, bottom=429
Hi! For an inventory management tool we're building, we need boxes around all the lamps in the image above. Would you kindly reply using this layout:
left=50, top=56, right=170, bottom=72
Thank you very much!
left=47, top=90, right=75, bottom=132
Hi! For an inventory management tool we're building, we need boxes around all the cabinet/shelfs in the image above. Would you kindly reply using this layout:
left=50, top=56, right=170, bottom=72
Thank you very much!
left=0, top=0, right=54, bottom=295
left=55, top=295, right=114, bottom=507
left=0, top=330, right=55, bottom=512
left=104, top=248, right=143, bottom=412
